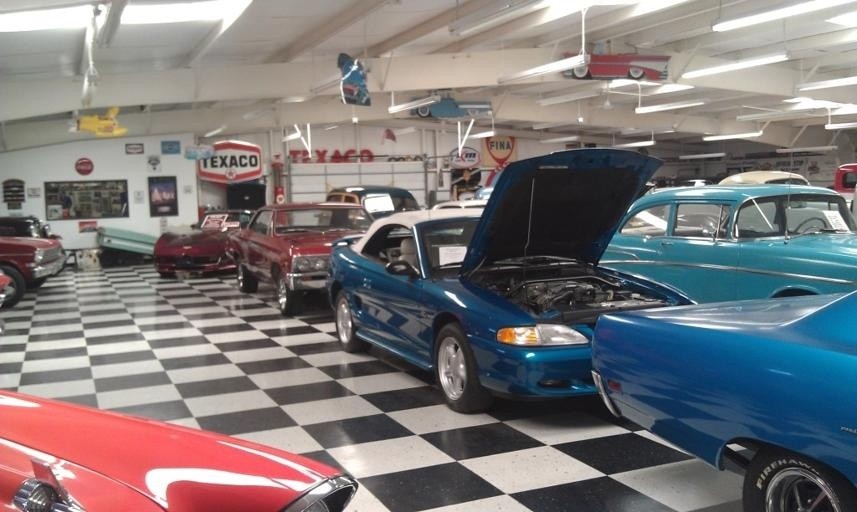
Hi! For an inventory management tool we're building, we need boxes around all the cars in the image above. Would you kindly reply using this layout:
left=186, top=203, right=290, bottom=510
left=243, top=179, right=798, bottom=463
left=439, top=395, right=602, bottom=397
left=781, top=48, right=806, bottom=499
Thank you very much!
left=1, top=215, right=64, bottom=303
left=835, top=161, right=857, bottom=184
left=599, top=180, right=857, bottom=298
left=322, top=147, right=701, bottom=418
left=1, top=379, right=366, bottom=510
left=724, top=172, right=808, bottom=192
left=238, top=204, right=375, bottom=317
left=595, top=285, right=856, bottom=507
left=327, top=185, right=419, bottom=221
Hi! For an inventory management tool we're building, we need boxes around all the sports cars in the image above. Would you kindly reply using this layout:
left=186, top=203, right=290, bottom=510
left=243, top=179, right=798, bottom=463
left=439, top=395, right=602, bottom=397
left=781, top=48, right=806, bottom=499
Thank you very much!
left=153, top=199, right=242, bottom=278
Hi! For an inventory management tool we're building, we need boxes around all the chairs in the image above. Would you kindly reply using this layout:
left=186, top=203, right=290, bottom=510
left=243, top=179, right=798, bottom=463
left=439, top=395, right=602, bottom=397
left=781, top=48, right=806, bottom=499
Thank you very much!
left=400, top=227, right=474, bottom=269
